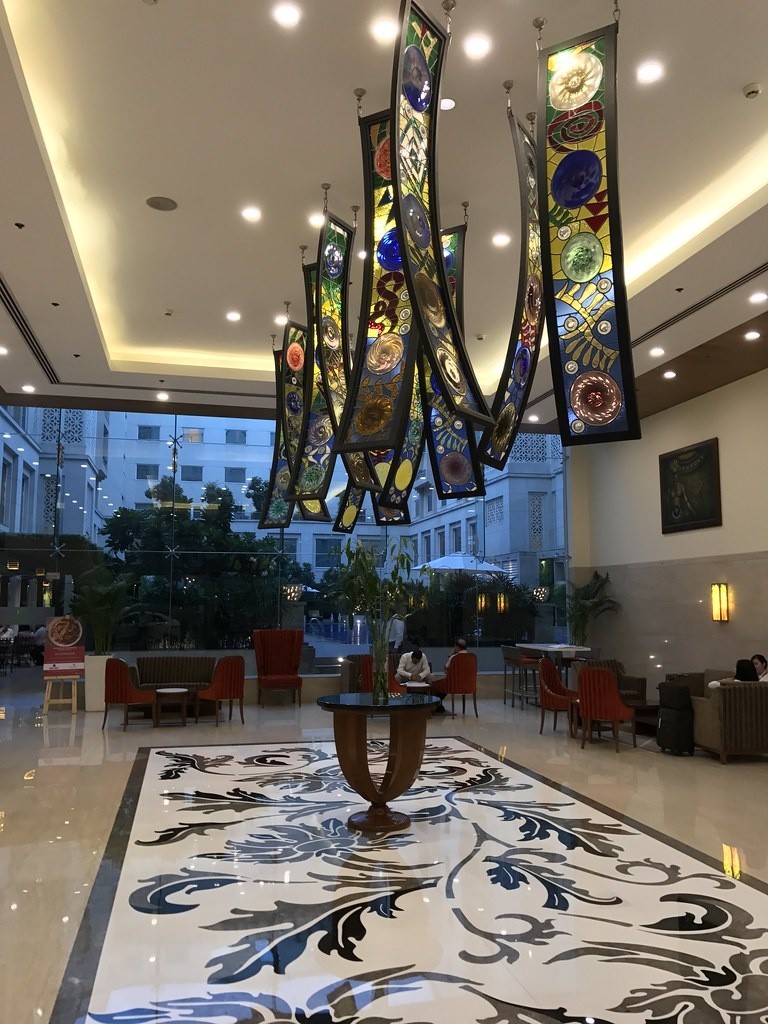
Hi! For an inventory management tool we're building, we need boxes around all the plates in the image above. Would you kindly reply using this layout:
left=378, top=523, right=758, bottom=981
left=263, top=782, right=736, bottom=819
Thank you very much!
left=48, top=617, right=82, bottom=648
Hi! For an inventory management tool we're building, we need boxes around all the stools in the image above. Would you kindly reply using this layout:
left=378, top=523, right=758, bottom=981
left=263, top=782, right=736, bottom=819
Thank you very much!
left=41, top=675, right=82, bottom=714
left=501, top=644, right=541, bottom=710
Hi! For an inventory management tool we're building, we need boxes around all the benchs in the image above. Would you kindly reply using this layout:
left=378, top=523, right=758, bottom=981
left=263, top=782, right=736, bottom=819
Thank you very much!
left=339, top=654, right=402, bottom=694
left=127, top=655, right=219, bottom=718
left=570, top=658, right=647, bottom=725
left=689, top=680, right=768, bottom=764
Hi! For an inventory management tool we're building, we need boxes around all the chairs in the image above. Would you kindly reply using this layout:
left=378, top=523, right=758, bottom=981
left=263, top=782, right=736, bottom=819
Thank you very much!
left=253, top=628, right=306, bottom=707
left=537, top=656, right=578, bottom=733
left=433, top=652, right=479, bottom=719
left=577, top=667, right=638, bottom=754
left=361, top=655, right=405, bottom=694
left=196, top=655, right=245, bottom=726
left=102, top=657, right=157, bottom=731
left=0, top=635, right=37, bottom=678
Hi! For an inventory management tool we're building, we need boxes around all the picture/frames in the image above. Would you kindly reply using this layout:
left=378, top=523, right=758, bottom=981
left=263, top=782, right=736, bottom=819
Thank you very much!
left=657, top=436, right=722, bottom=534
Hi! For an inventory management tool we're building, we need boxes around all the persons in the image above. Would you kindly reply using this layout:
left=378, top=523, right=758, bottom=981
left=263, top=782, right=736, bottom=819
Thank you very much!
left=0, top=625, right=13, bottom=643
left=394, top=649, right=433, bottom=685
left=30, top=625, right=46, bottom=666
left=386, top=613, right=403, bottom=654
left=431, top=639, right=467, bottom=713
left=708, top=659, right=759, bottom=689
left=751, top=654, right=768, bottom=681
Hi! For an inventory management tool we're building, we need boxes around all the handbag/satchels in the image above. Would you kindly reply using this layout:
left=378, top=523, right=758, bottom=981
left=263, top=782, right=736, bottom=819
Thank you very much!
left=658, top=682, right=691, bottom=708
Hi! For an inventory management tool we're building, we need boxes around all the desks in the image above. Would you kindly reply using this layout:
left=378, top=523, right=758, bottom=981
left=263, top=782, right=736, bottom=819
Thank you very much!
left=399, top=682, right=431, bottom=695
left=624, top=696, right=661, bottom=736
left=517, top=642, right=591, bottom=688
left=155, top=688, right=189, bottom=725
left=317, top=689, right=442, bottom=833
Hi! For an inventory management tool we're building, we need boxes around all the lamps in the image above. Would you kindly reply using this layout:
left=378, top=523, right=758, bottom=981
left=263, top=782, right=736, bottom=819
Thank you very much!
left=711, top=582, right=729, bottom=622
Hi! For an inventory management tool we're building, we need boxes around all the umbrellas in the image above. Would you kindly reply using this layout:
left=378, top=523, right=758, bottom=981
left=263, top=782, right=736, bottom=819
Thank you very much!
left=302, top=584, right=320, bottom=592
left=410, top=551, right=512, bottom=575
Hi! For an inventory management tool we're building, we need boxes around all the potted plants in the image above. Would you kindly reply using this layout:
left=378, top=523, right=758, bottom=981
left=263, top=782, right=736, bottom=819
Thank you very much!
left=324, top=532, right=437, bottom=703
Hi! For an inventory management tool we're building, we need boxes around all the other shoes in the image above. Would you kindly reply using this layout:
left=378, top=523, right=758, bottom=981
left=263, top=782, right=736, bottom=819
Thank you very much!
left=436, top=705, right=445, bottom=712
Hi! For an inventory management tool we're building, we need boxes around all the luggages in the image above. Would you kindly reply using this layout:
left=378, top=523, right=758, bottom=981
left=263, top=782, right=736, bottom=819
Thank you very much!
left=657, top=706, right=694, bottom=756
